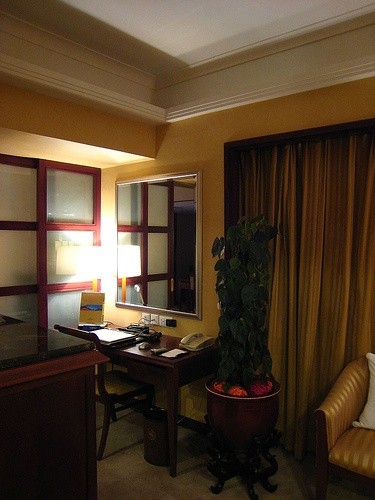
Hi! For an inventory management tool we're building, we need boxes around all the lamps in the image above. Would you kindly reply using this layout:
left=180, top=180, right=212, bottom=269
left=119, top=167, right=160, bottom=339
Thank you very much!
left=55, top=243, right=112, bottom=331
left=115, top=241, right=142, bottom=305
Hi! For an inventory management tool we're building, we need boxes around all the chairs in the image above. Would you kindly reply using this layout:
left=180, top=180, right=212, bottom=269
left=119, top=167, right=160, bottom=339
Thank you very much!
left=53, top=324, right=154, bottom=462
left=311, top=355, right=375, bottom=500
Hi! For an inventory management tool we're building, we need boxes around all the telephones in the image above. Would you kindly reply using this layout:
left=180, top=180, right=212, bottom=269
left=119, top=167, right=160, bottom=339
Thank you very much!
left=180, top=331, right=213, bottom=351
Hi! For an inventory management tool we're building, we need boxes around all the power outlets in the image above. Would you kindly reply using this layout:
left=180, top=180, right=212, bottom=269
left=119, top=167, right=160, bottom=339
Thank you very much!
left=142, top=312, right=177, bottom=330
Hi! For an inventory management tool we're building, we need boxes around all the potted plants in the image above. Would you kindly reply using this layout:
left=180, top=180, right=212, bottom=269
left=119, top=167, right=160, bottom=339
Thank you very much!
left=203, top=215, right=283, bottom=444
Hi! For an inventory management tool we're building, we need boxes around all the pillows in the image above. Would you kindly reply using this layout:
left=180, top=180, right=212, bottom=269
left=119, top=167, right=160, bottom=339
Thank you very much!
left=352, top=352, right=375, bottom=431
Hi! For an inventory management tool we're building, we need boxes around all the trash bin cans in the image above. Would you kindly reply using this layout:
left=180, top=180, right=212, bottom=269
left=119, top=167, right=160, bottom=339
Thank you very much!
left=143, top=405, right=170, bottom=466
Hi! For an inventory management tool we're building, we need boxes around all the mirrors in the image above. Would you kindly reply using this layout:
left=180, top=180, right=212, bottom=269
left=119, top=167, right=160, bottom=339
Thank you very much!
left=113, top=167, right=207, bottom=321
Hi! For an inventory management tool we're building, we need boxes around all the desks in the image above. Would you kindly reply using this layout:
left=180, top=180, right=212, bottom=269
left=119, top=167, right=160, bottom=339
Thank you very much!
left=52, top=322, right=218, bottom=479
